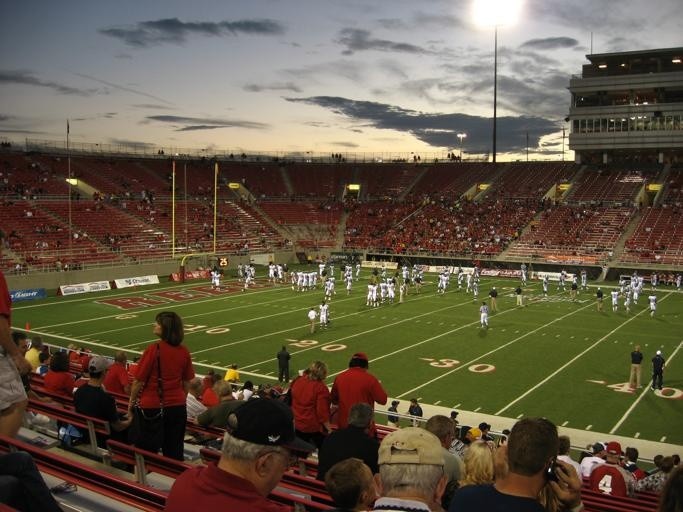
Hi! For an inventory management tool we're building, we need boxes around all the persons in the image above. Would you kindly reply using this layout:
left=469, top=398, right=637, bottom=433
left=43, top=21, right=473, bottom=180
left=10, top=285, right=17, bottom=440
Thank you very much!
left=428, top=153, right=683, bottom=328
left=380, top=380, right=682, bottom=512
left=1, top=150, right=427, bottom=335
left=648, top=348, right=666, bottom=390
left=574, top=94, right=683, bottom=165
left=627, top=343, right=642, bottom=389
left=0, top=336, right=378, bottom=510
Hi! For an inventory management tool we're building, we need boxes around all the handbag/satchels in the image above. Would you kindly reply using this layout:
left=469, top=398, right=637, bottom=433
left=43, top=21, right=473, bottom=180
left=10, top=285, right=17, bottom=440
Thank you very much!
left=127, top=406, right=166, bottom=445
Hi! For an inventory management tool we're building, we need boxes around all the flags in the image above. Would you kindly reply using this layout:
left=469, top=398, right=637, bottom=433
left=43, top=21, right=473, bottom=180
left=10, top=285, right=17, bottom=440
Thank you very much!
left=66, top=120, right=69, bottom=135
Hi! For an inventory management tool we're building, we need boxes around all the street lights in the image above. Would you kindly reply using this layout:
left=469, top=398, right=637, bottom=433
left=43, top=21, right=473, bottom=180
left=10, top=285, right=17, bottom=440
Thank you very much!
left=457, top=133, right=466, bottom=162
left=466, top=0, right=524, bottom=161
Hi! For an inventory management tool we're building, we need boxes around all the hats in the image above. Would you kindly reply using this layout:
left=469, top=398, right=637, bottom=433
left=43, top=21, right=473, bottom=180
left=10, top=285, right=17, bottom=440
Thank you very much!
left=88, top=356, right=113, bottom=372
left=607, top=442, right=622, bottom=456
left=352, top=352, right=368, bottom=360
left=378, top=427, right=444, bottom=465
left=226, top=398, right=315, bottom=452
left=389, top=399, right=511, bottom=435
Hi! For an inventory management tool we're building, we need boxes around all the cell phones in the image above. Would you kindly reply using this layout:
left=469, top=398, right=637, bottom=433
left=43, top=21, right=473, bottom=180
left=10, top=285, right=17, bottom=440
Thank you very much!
left=547, top=457, right=561, bottom=484
